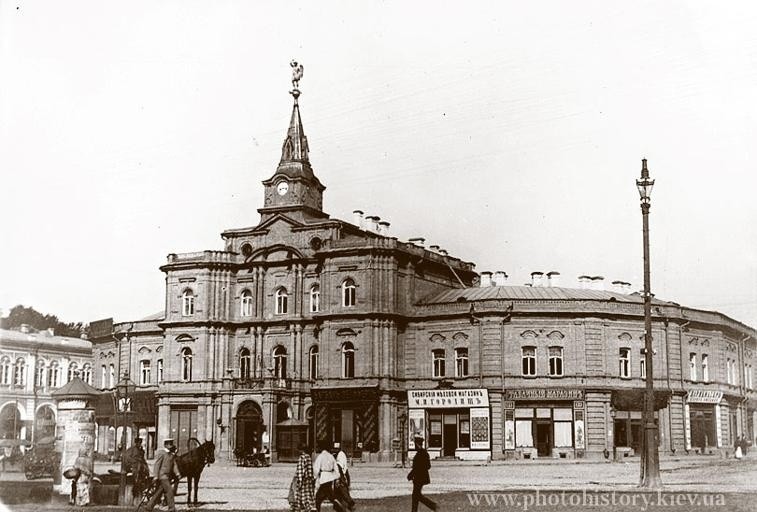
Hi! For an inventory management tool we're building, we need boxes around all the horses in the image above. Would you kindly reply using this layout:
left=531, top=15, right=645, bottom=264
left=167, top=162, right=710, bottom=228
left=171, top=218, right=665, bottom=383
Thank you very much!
left=173, top=438, right=216, bottom=508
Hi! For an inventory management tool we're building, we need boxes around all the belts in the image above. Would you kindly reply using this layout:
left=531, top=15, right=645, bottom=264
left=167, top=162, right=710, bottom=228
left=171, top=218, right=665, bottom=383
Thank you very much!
left=320, top=470, right=334, bottom=472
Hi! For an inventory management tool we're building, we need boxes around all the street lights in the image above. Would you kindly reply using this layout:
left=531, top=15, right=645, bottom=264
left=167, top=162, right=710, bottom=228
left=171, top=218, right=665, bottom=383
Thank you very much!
left=636, top=156, right=663, bottom=489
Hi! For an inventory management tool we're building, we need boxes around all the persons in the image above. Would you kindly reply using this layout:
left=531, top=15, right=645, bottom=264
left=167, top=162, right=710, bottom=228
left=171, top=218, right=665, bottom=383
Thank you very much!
left=64, top=430, right=185, bottom=511
left=740, top=433, right=750, bottom=456
left=407, top=435, right=437, bottom=512
left=734, top=435, right=742, bottom=459
left=261, top=444, right=270, bottom=464
left=330, top=442, right=357, bottom=511
left=312, top=440, right=347, bottom=511
left=288, top=442, right=315, bottom=512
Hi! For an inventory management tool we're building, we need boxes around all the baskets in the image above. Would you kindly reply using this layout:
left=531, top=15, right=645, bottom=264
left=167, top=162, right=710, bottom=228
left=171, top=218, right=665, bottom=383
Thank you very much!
left=63, top=468, right=80, bottom=478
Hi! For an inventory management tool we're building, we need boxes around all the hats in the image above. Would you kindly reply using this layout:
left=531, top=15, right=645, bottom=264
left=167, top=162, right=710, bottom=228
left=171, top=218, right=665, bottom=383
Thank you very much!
left=414, top=436, right=424, bottom=443
left=164, top=438, right=174, bottom=444
left=315, top=439, right=330, bottom=447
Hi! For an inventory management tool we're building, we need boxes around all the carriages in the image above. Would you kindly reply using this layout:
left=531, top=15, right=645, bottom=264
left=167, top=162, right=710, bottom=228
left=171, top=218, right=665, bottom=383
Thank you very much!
left=232, top=447, right=271, bottom=467
left=93, top=437, right=216, bottom=508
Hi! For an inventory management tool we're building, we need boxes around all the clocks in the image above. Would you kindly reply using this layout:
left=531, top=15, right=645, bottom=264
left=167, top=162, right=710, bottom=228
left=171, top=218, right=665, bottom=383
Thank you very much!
left=277, top=180, right=289, bottom=196
